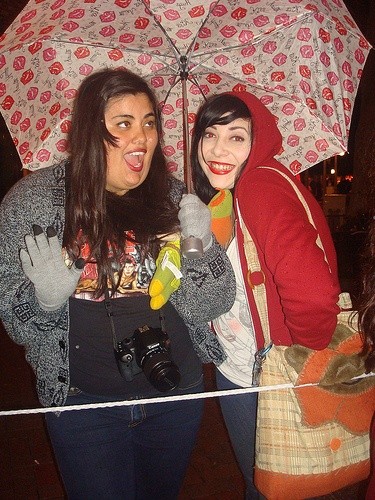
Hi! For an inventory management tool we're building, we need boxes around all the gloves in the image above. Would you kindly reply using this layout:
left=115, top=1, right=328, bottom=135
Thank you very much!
left=178, top=193, right=214, bottom=253
left=20, top=223, right=86, bottom=312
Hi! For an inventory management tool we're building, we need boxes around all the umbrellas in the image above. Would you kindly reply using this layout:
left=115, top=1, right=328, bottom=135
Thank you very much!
left=0, top=0, right=373, bottom=183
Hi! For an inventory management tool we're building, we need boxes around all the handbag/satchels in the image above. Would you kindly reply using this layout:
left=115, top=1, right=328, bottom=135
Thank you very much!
left=252, top=310, right=375, bottom=500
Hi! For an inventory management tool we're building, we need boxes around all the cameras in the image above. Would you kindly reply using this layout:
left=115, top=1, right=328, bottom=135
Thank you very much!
left=114, top=325, right=181, bottom=392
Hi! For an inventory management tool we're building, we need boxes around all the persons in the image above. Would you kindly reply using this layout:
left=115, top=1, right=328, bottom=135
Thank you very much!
left=183, top=86, right=346, bottom=499
left=0, top=67, right=240, bottom=500
